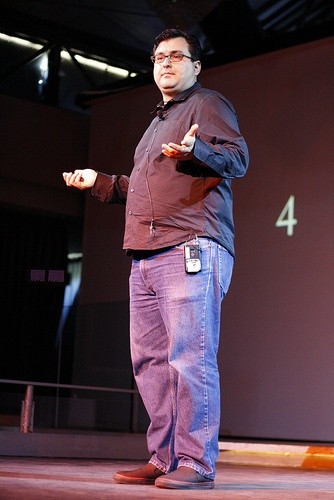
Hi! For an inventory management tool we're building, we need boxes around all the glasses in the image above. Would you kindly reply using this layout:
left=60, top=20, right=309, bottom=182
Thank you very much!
left=150, top=51, right=195, bottom=63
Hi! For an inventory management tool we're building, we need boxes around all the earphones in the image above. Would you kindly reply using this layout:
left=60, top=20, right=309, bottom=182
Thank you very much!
left=195, top=66, right=197, bottom=68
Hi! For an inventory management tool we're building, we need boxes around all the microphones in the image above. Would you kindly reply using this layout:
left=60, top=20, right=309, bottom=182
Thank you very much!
left=156, top=110, right=163, bottom=121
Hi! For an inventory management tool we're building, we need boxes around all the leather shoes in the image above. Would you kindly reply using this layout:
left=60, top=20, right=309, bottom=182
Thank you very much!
left=113, top=451, right=215, bottom=490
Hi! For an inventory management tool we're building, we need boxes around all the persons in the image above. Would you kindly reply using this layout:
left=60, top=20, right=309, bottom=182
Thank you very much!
left=62, top=28, right=250, bottom=490
